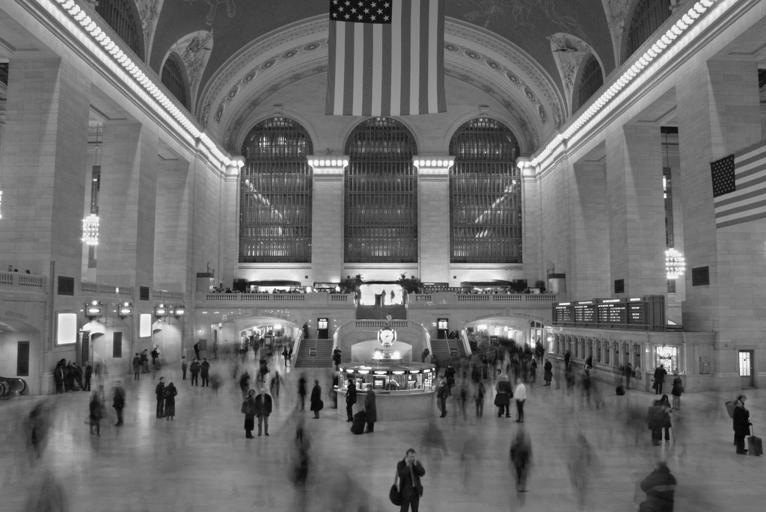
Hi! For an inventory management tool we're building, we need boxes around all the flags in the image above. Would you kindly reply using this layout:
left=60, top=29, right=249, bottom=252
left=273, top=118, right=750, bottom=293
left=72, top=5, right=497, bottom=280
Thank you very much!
left=324, top=0, right=448, bottom=116
left=710, top=138, right=766, bottom=227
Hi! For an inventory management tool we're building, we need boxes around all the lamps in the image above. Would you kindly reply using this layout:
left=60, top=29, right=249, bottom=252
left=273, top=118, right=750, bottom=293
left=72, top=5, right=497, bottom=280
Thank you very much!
left=665, top=128, right=686, bottom=280
left=81, top=121, right=100, bottom=245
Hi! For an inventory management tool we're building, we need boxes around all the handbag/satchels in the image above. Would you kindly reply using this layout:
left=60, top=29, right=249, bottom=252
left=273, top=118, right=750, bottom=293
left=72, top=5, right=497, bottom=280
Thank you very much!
left=390, top=484, right=402, bottom=505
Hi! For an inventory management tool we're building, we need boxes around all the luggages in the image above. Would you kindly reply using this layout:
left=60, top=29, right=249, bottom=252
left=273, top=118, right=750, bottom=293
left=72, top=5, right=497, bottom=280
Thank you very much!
left=748, top=424, right=762, bottom=456
left=351, top=411, right=366, bottom=434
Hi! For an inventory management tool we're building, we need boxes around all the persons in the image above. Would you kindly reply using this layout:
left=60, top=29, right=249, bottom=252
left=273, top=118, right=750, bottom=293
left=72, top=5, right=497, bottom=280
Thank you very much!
left=396, top=448, right=426, bottom=512
left=156, top=376, right=177, bottom=421
left=421, top=333, right=683, bottom=446
left=424, top=286, right=532, bottom=300
left=731, top=395, right=756, bottom=454
left=232, top=329, right=376, bottom=498
left=639, top=459, right=678, bottom=512
left=132, top=343, right=161, bottom=380
left=89, top=391, right=103, bottom=437
left=54, top=358, right=92, bottom=391
left=180, top=357, right=209, bottom=387
left=508, top=428, right=530, bottom=493
left=213, top=286, right=301, bottom=299
left=111, top=389, right=124, bottom=427
left=193, top=343, right=202, bottom=360
